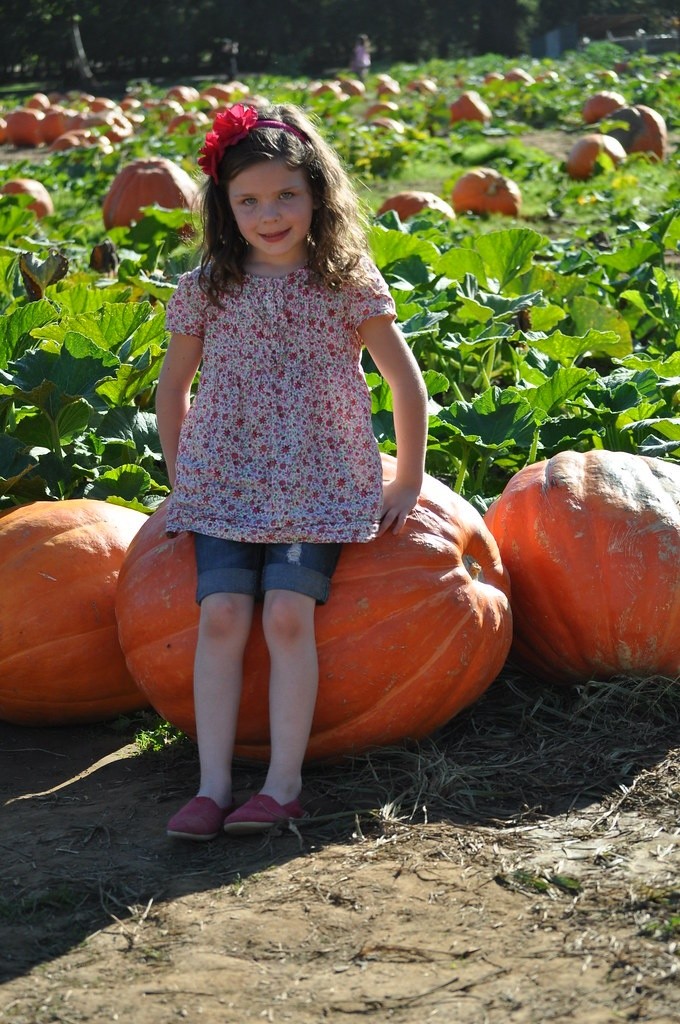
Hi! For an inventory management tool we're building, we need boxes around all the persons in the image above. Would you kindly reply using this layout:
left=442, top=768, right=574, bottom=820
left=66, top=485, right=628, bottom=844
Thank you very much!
left=156, top=95, right=430, bottom=838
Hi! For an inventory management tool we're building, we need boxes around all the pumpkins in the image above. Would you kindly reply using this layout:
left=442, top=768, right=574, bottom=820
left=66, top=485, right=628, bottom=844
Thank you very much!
left=116, top=449, right=512, bottom=762
left=480, top=450, right=680, bottom=687
left=0, top=499, right=151, bottom=725
left=0, top=66, right=667, bottom=241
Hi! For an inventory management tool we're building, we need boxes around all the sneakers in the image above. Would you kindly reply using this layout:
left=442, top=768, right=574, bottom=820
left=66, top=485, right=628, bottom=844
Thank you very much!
left=165, top=794, right=237, bottom=840
left=222, top=793, right=307, bottom=836
